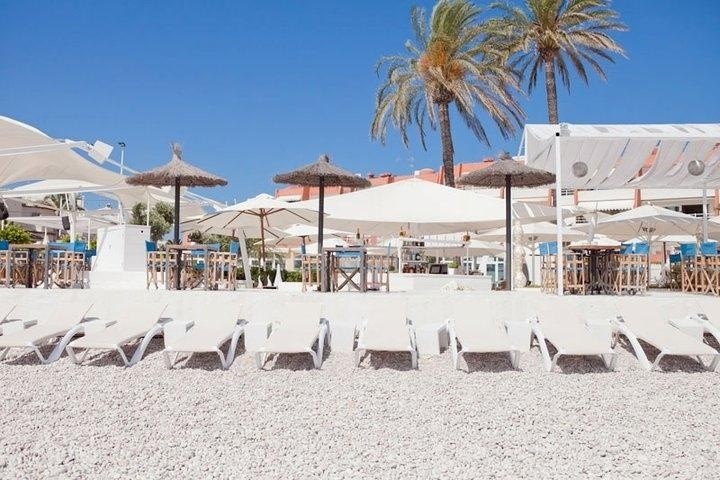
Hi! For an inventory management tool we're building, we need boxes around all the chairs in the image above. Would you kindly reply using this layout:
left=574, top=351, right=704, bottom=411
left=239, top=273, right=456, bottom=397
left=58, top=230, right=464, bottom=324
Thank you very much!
left=0, top=293, right=720, bottom=373
left=145, top=238, right=389, bottom=292
left=0, top=239, right=96, bottom=290
left=539, top=239, right=720, bottom=295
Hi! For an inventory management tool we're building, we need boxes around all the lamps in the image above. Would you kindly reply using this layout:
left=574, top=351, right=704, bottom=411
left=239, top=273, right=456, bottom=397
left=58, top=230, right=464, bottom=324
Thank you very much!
left=687, top=146, right=705, bottom=176
left=571, top=149, right=588, bottom=178
